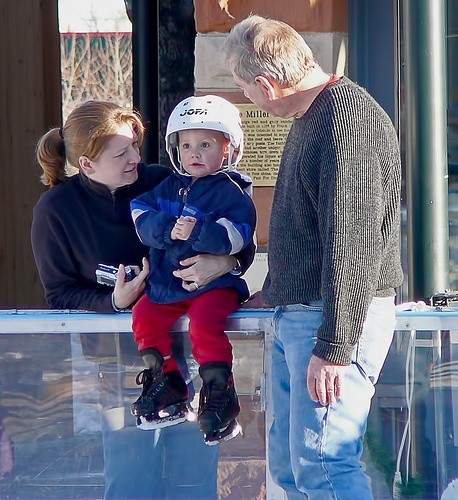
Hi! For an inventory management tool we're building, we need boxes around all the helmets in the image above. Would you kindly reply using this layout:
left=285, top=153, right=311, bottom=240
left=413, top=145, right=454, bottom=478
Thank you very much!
left=165, top=94, right=244, bottom=153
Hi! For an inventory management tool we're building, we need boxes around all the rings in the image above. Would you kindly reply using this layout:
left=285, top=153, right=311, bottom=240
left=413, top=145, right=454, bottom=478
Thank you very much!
left=194, top=282, right=198, bottom=289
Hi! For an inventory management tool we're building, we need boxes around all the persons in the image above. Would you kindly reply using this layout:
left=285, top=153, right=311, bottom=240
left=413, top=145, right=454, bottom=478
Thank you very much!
left=224, top=16, right=403, bottom=500
left=130, top=95, right=257, bottom=446
left=32, top=101, right=258, bottom=499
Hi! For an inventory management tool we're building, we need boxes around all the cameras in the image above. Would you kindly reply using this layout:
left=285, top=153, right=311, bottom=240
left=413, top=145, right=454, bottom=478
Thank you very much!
left=95, top=263, right=133, bottom=287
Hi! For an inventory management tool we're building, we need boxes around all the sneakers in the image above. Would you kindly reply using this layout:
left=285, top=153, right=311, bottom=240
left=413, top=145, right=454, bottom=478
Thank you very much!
left=130, top=348, right=195, bottom=430
left=198, top=362, right=242, bottom=447
